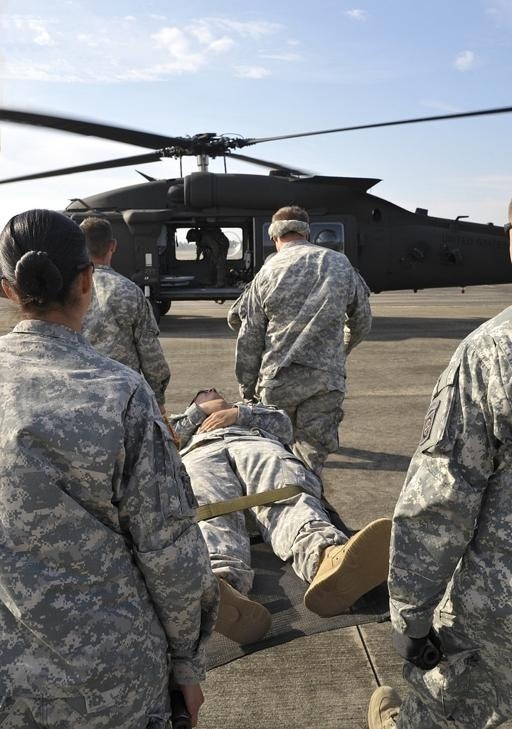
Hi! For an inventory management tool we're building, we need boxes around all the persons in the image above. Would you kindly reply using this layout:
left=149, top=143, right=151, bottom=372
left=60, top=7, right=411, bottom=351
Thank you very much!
left=185, top=228, right=227, bottom=291
left=0, top=208, right=222, bottom=728
left=227, top=252, right=276, bottom=396
left=233, top=205, right=374, bottom=481
left=77, top=218, right=172, bottom=421
left=166, top=386, right=399, bottom=649
left=366, top=197, right=512, bottom=728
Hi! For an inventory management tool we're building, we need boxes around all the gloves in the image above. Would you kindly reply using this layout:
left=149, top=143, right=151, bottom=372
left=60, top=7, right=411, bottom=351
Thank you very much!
left=390, top=625, right=441, bottom=671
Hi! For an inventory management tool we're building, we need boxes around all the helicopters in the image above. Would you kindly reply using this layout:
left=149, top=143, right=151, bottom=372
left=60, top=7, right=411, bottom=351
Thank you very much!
left=1, top=105, right=512, bottom=326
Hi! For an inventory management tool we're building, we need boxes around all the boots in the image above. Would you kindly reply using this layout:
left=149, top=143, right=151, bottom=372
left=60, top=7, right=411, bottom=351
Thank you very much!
left=368, top=685, right=405, bottom=729
left=303, top=515, right=392, bottom=615
left=210, top=574, right=272, bottom=645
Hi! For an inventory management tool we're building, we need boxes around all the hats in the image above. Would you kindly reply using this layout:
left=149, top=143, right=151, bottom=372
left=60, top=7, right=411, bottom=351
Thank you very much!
left=186, top=229, right=194, bottom=242
